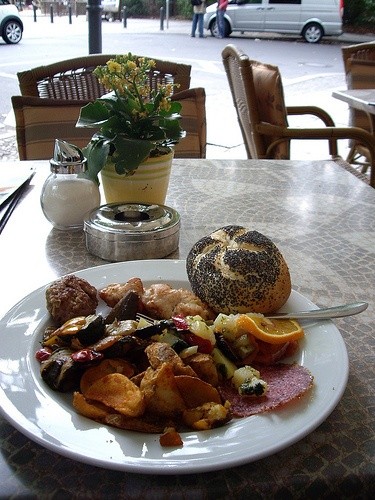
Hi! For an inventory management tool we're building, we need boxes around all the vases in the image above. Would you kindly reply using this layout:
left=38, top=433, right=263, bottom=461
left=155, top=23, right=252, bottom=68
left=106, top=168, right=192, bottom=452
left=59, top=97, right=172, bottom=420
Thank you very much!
left=101, top=148, right=175, bottom=207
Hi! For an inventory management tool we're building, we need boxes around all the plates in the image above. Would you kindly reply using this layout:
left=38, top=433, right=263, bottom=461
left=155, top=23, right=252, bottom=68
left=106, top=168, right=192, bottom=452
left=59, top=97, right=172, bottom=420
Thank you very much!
left=1, top=260, right=350, bottom=474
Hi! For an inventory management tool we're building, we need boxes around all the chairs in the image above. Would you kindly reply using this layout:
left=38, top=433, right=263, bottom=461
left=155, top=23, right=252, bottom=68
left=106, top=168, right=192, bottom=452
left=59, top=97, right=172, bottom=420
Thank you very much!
left=17, top=53, right=191, bottom=101
left=341, top=42, right=375, bottom=175
left=221, top=44, right=375, bottom=188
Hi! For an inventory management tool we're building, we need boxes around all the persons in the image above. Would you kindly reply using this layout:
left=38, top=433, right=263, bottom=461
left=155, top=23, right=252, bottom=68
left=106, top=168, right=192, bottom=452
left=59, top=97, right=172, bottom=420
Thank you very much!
left=191, top=0, right=207, bottom=38
left=216, top=0, right=228, bottom=39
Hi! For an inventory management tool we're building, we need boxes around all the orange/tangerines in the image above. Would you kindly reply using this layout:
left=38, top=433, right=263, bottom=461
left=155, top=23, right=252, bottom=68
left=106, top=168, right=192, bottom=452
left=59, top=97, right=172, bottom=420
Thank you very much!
left=236, top=313, right=303, bottom=344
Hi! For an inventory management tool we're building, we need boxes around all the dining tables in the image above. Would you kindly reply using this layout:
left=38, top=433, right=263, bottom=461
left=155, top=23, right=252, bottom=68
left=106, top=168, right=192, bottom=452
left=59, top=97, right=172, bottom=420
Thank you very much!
left=0, top=157, right=375, bottom=500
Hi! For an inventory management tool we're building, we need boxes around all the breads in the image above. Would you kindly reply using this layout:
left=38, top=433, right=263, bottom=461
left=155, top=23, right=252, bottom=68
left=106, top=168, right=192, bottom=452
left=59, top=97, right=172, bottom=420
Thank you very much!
left=186, top=224, right=292, bottom=315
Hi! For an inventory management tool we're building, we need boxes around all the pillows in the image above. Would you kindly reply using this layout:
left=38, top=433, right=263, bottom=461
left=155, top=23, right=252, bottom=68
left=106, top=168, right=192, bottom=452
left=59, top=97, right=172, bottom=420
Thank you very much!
left=11, top=86, right=206, bottom=160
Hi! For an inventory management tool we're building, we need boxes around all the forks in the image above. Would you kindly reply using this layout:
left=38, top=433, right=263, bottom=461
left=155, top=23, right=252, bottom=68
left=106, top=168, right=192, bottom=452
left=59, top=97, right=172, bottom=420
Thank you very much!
left=135, top=302, right=369, bottom=329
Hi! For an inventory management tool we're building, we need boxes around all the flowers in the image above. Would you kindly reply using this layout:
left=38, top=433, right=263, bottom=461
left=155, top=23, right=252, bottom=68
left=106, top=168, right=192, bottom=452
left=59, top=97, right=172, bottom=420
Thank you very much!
left=75, top=52, right=187, bottom=186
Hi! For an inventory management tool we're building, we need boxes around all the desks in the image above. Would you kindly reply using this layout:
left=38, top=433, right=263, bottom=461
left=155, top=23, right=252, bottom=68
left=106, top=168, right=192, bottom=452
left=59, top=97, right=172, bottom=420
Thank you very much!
left=332, top=89, right=375, bottom=133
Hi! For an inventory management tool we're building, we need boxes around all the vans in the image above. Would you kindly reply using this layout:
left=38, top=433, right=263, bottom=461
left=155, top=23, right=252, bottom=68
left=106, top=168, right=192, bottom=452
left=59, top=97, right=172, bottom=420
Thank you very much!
left=203, top=0, right=344, bottom=43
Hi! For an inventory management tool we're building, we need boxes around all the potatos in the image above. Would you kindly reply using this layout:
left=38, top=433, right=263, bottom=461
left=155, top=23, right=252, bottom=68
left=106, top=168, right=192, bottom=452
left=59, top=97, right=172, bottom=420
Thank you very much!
left=105, top=312, right=269, bottom=399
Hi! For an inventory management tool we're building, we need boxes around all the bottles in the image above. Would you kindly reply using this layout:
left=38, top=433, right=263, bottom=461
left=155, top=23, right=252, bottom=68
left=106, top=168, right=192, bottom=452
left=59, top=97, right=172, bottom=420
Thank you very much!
left=40, top=138, right=101, bottom=230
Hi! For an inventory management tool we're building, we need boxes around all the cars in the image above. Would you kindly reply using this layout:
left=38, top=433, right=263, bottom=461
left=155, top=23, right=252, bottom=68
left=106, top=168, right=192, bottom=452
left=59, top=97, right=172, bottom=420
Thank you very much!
left=0, top=0, right=23, bottom=44
